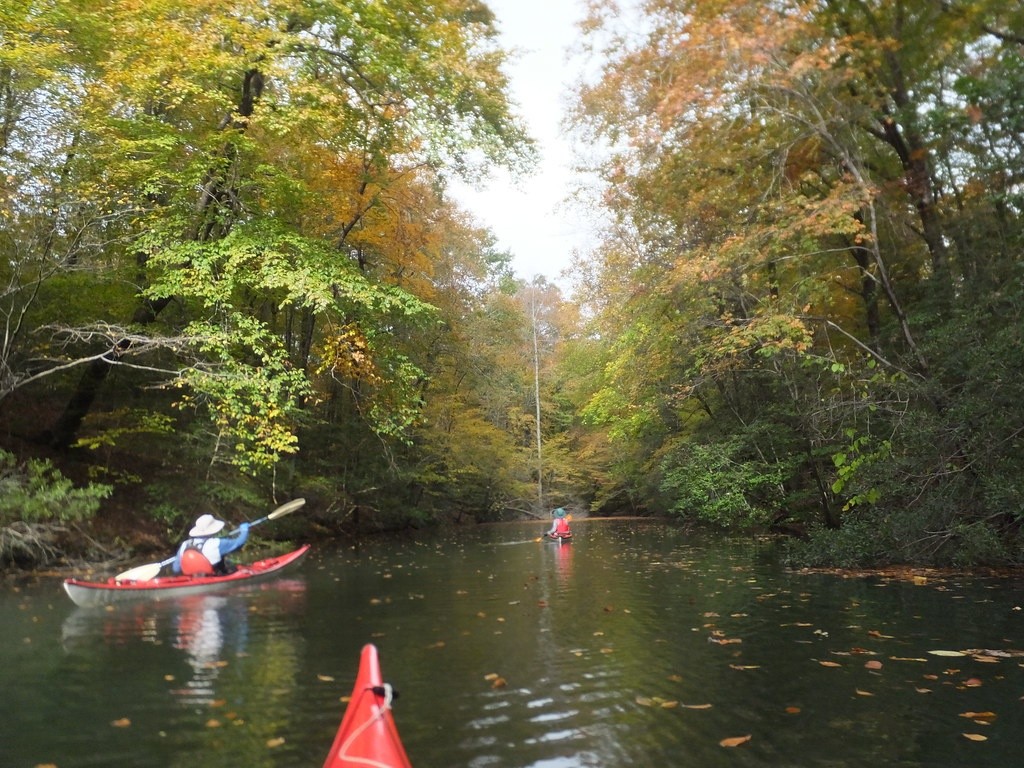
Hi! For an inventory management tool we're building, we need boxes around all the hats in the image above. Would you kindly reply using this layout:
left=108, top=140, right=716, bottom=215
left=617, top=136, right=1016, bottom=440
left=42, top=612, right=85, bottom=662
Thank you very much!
left=189, top=514, right=224, bottom=537
left=554, top=508, right=566, bottom=518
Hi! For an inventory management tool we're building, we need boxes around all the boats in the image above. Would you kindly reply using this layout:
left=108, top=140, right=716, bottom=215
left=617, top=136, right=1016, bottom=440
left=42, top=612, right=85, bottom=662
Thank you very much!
left=62, top=544, right=312, bottom=609
left=548, top=532, right=572, bottom=547
left=322, top=643, right=413, bottom=768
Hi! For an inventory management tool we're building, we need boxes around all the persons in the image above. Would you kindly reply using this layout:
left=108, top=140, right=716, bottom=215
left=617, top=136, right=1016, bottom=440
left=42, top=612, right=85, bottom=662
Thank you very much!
left=547, top=508, right=570, bottom=535
left=173, top=514, right=249, bottom=576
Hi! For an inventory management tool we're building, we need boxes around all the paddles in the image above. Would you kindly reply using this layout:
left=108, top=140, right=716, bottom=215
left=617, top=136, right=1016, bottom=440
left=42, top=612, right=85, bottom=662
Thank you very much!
left=114, top=497, right=306, bottom=581
left=534, top=514, right=573, bottom=543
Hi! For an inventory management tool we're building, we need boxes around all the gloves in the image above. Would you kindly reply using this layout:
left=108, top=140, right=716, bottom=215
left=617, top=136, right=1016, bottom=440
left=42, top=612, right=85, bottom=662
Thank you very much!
left=240, top=522, right=249, bottom=532
